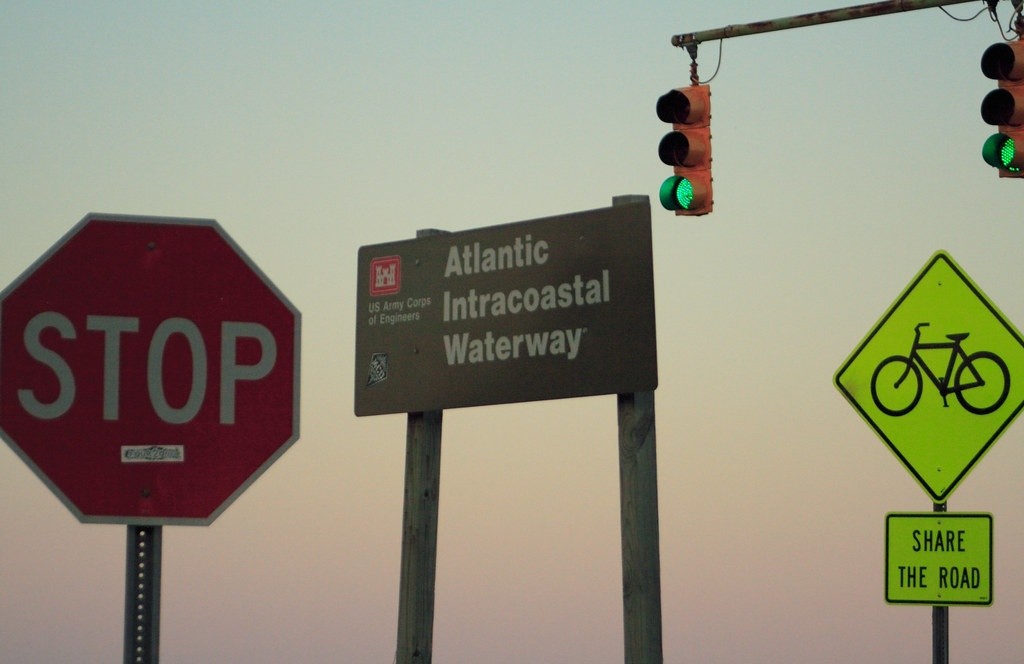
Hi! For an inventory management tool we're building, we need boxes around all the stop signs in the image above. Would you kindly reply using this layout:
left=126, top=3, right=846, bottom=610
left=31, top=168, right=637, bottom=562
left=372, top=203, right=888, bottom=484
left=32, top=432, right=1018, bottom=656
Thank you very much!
left=1, top=215, right=300, bottom=529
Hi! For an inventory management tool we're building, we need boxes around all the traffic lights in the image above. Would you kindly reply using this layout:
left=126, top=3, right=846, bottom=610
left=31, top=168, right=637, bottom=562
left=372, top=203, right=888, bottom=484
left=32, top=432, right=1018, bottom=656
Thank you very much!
left=654, top=81, right=716, bottom=216
left=979, top=40, right=1024, bottom=177
